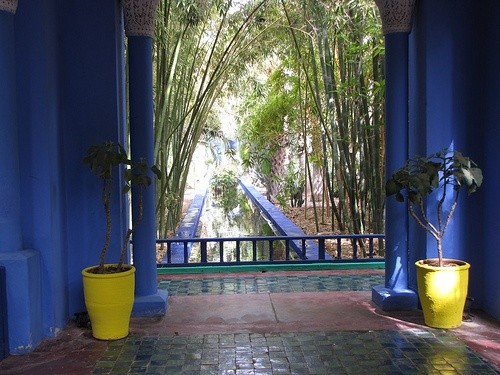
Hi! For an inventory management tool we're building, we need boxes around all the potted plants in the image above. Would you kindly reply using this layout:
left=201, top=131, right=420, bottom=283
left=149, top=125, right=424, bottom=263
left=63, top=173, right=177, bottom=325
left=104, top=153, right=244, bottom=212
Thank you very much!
left=80, top=139, right=164, bottom=342
left=385, top=152, right=484, bottom=332
